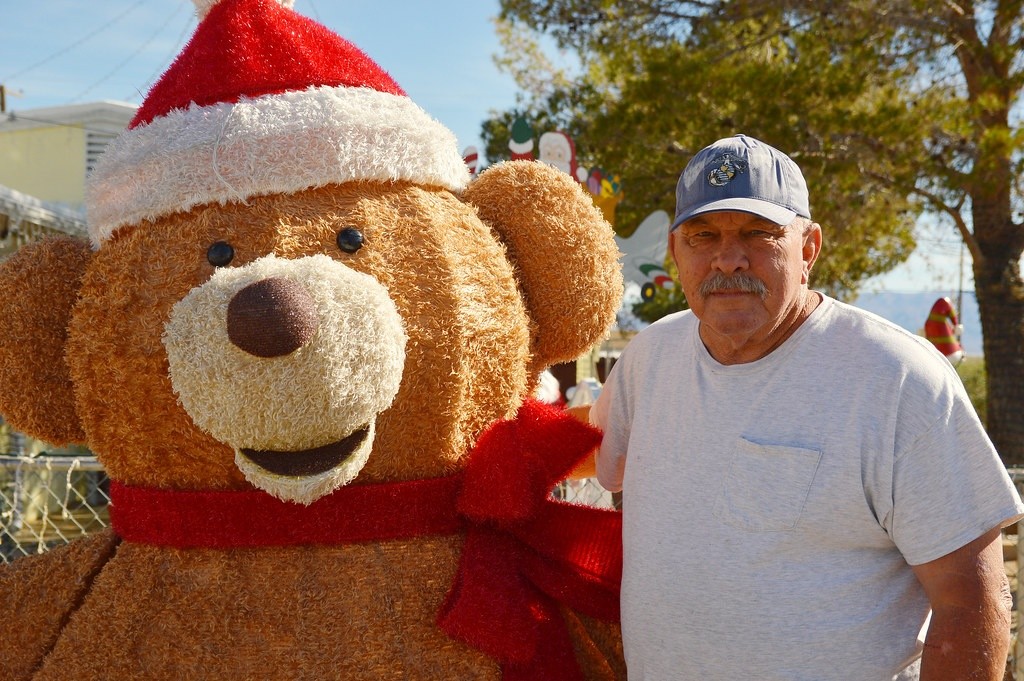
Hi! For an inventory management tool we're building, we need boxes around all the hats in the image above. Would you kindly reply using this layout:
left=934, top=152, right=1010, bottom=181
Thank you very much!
left=669, top=134, right=815, bottom=226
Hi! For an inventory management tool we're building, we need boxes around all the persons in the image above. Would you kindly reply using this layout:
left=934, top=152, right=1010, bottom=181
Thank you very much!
left=549, top=131, right=1024, bottom=681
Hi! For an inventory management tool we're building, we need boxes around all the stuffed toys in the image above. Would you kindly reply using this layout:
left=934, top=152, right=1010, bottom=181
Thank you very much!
left=0, top=0, right=624, bottom=681
